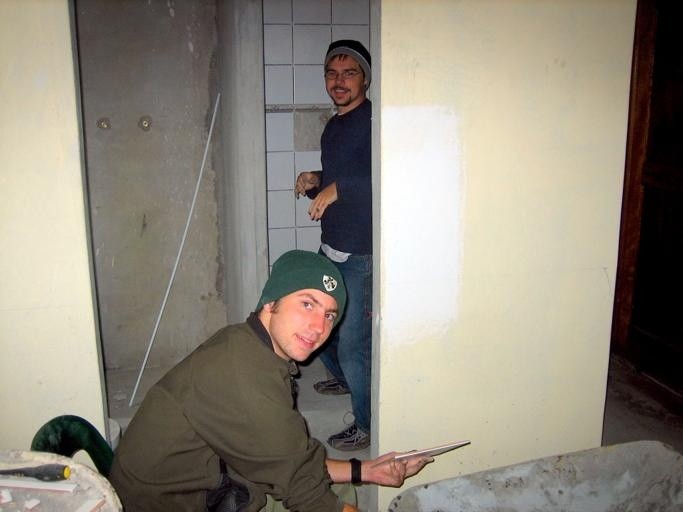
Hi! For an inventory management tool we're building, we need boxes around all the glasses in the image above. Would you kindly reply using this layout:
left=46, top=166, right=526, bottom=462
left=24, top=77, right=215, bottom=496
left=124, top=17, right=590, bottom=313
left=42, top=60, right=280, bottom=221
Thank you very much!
left=322, top=67, right=364, bottom=81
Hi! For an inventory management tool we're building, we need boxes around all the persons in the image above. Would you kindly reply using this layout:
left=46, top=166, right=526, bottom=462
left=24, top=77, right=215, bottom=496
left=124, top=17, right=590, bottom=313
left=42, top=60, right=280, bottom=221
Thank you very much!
left=102, top=247, right=437, bottom=512
left=291, top=37, right=377, bottom=452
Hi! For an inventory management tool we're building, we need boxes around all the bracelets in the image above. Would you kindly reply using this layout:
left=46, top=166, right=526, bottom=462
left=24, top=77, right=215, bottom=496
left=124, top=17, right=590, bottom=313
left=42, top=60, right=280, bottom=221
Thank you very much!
left=348, top=457, right=361, bottom=483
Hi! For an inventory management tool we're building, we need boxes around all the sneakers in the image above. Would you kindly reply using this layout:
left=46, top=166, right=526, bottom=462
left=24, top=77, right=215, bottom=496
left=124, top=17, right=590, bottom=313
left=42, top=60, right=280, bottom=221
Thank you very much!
left=312, top=377, right=349, bottom=395
left=325, top=421, right=370, bottom=452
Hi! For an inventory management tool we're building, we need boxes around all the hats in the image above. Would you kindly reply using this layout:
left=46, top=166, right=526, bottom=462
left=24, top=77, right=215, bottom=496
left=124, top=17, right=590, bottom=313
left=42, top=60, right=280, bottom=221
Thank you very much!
left=252, top=249, right=348, bottom=329
left=323, top=38, right=371, bottom=91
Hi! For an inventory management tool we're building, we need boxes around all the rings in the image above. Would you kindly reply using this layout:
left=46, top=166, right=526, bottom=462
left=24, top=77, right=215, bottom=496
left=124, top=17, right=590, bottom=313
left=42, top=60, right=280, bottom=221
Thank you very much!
left=316, top=208, right=320, bottom=211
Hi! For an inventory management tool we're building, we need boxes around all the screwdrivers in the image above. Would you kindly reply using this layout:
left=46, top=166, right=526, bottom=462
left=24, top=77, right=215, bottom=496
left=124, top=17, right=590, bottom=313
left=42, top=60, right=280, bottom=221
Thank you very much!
left=0, top=464, right=70, bottom=482
